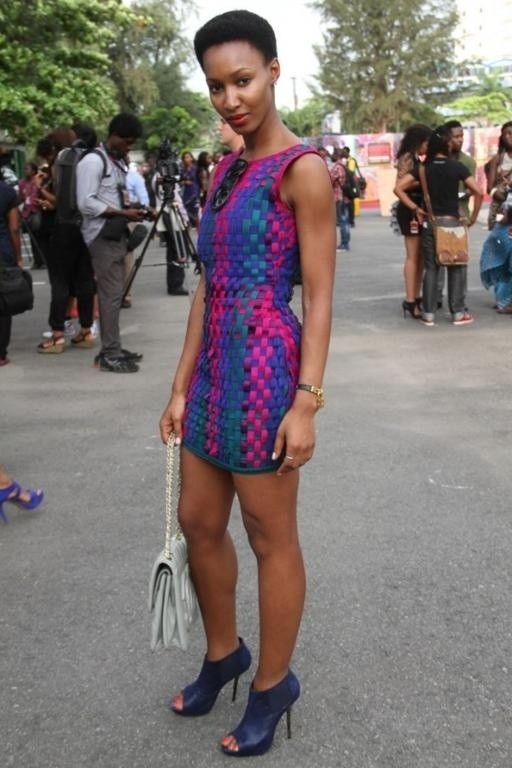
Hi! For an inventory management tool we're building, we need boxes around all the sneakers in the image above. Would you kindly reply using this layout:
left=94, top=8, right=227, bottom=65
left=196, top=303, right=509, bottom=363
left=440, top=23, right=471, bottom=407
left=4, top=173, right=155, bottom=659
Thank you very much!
left=402, top=298, right=512, bottom=326
left=168, top=287, right=189, bottom=295
left=38, top=320, right=141, bottom=371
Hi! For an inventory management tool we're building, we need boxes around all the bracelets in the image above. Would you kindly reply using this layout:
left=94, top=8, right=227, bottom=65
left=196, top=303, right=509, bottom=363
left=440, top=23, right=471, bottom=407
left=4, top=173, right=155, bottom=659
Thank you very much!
left=294, top=382, right=327, bottom=412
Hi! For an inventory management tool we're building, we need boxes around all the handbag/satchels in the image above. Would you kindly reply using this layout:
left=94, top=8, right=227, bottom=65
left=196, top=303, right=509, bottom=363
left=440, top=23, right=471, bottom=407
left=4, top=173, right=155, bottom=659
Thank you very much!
left=22, top=212, right=42, bottom=232
left=432, top=225, right=468, bottom=266
left=146, top=535, right=200, bottom=652
left=358, top=176, right=366, bottom=190
left=0, top=265, right=33, bottom=314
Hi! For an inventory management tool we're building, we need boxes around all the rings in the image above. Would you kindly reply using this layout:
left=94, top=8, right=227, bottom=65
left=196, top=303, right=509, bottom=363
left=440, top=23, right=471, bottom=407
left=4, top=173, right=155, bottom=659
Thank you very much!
left=286, top=454, right=293, bottom=461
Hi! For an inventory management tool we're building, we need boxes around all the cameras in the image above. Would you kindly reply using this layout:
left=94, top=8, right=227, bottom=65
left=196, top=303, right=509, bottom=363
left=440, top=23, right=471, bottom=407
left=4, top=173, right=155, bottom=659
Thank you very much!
left=134, top=202, right=155, bottom=222
left=161, top=175, right=176, bottom=200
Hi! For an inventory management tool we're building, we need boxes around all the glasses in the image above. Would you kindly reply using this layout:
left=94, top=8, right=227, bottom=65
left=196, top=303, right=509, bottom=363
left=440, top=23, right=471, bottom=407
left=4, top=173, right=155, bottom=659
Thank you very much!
left=211, top=159, right=247, bottom=210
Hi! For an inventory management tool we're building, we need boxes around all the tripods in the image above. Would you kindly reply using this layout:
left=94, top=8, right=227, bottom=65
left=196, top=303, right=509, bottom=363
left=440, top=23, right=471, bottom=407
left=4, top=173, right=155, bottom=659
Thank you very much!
left=121, top=198, right=202, bottom=308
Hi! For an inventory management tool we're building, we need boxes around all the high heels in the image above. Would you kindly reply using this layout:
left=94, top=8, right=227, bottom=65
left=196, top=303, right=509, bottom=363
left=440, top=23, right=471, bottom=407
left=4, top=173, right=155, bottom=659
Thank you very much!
left=221, top=670, right=300, bottom=756
left=170, top=636, right=250, bottom=716
left=1, top=479, right=44, bottom=523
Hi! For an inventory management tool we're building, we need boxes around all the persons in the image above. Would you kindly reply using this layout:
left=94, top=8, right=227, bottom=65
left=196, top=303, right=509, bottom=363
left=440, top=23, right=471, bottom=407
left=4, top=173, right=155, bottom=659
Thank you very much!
left=317, top=146, right=356, bottom=250
left=0, top=455, right=48, bottom=526
left=392, top=118, right=512, bottom=327
left=157, top=10, right=338, bottom=759
left=1, top=113, right=223, bottom=373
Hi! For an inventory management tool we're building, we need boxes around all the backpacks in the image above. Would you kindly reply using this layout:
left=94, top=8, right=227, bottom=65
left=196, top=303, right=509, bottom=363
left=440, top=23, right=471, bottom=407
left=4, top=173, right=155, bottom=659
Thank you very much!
left=51, top=139, right=108, bottom=225
left=338, top=162, right=361, bottom=198
